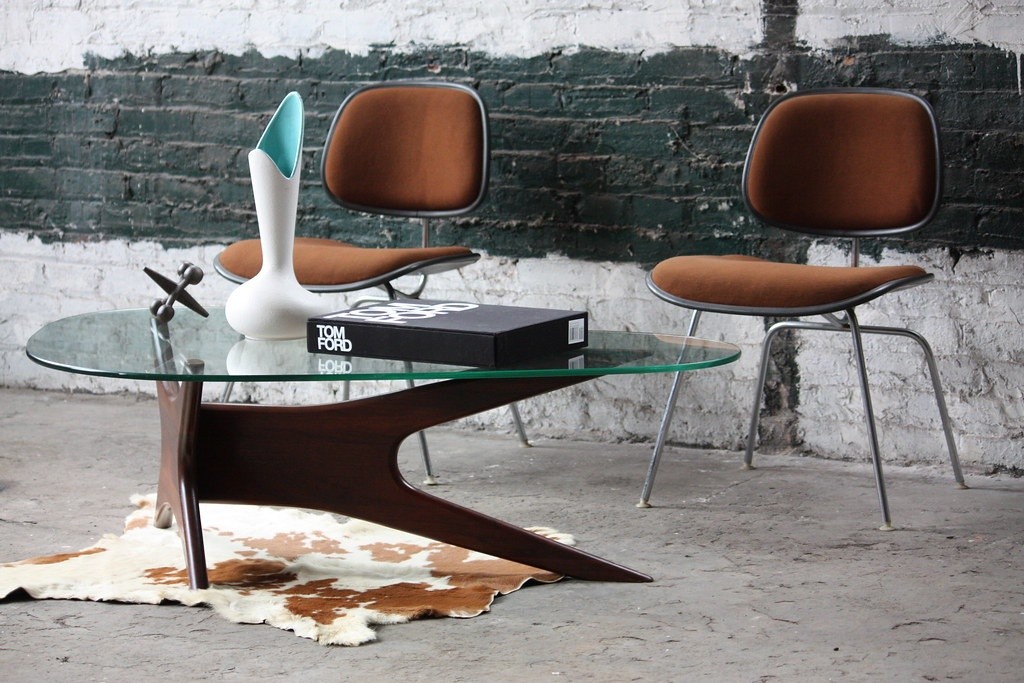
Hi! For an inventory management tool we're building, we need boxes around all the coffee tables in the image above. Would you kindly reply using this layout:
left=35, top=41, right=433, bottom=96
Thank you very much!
left=26, top=304, right=742, bottom=589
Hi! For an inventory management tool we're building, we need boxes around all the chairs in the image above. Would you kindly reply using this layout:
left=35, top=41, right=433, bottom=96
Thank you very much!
left=636, top=87, right=969, bottom=535
left=213, top=81, right=534, bottom=487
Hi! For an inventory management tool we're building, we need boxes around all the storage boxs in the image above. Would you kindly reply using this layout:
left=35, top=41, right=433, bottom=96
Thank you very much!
left=307, top=299, right=588, bottom=370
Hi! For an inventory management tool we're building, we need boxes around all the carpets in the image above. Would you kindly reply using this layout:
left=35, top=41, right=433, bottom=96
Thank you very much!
left=0, top=491, right=577, bottom=644
